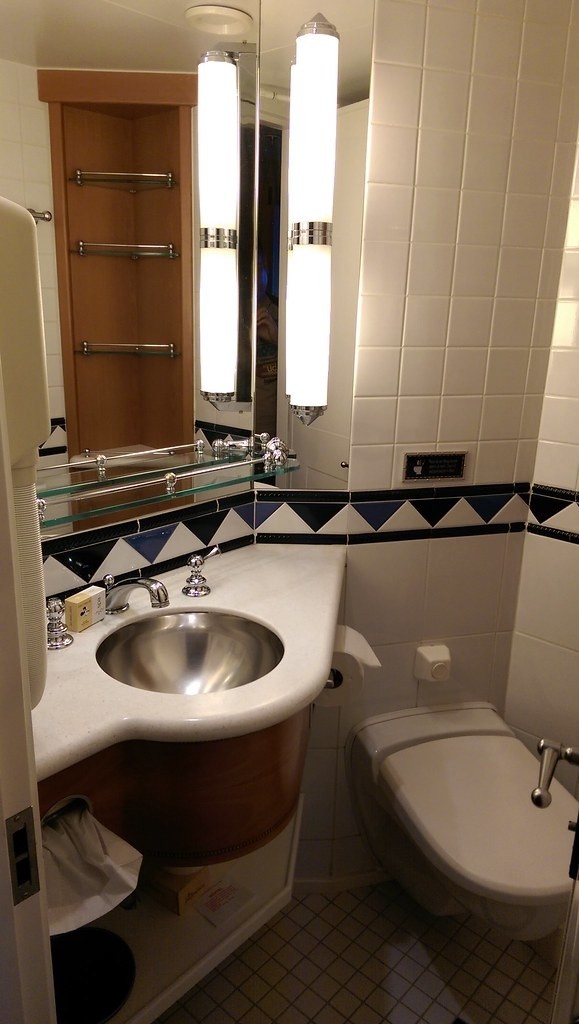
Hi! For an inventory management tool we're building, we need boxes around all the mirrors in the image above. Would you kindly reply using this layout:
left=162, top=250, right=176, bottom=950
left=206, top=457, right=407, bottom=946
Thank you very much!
left=0, top=1, right=257, bottom=544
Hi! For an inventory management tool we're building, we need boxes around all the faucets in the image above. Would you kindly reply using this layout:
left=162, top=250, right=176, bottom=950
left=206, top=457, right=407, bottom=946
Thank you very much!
left=104, top=576, right=171, bottom=616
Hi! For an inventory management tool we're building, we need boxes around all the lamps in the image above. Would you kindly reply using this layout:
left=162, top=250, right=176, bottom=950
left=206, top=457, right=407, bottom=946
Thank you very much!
left=285, top=13, right=341, bottom=425
left=196, top=50, right=240, bottom=402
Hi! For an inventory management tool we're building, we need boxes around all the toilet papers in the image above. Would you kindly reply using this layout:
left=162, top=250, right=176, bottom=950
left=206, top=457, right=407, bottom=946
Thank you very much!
left=309, top=624, right=383, bottom=708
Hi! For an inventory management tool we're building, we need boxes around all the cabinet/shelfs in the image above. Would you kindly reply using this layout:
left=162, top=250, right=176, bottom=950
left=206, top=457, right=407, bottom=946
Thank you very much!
left=36, top=69, right=199, bottom=467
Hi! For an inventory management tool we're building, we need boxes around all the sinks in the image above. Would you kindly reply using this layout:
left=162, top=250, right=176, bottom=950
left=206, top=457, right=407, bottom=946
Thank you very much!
left=93, top=601, right=287, bottom=700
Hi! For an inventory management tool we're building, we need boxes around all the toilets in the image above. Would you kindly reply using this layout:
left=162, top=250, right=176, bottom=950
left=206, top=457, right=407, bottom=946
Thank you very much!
left=344, top=697, right=579, bottom=948
